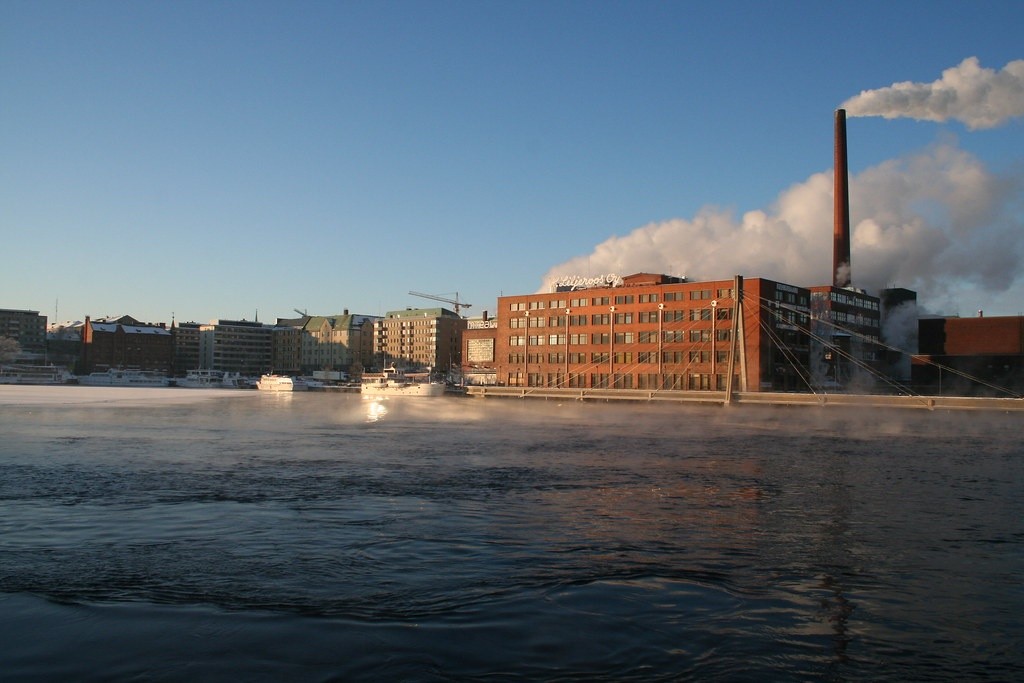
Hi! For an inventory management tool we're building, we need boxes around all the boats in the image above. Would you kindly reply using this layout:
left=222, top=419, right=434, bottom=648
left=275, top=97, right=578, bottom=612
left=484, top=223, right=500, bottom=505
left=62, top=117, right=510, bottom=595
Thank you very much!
left=255, top=366, right=309, bottom=392
left=360, top=376, right=445, bottom=396
left=0, top=359, right=341, bottom=389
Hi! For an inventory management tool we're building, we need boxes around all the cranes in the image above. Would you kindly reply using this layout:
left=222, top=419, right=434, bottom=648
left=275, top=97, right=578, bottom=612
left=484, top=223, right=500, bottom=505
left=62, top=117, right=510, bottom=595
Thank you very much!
left=408, top=289, right=473, bottom=314
left=293, top=307, right=309, bottom=317
